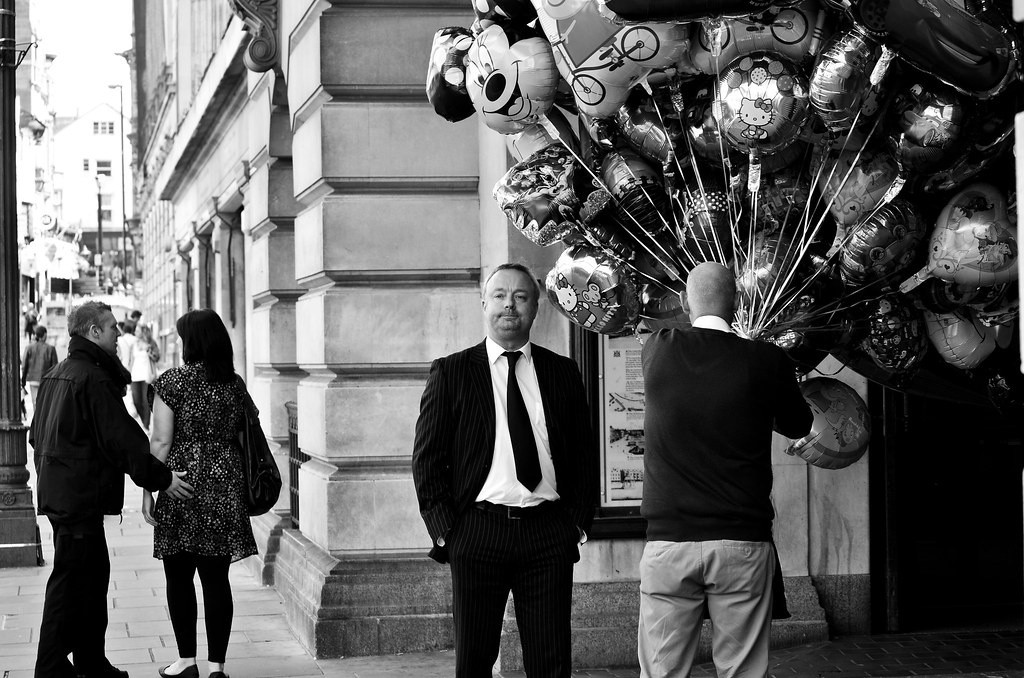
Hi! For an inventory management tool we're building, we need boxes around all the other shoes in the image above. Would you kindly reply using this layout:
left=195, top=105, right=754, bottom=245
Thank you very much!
left=208, top=671, right=229, bottom=678
left=159, top=663, right=199, bottom=678
left=34, top=658, right=129, bottom=678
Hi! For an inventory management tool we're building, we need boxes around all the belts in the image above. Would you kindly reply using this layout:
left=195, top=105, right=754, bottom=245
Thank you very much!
left=475, top=502, right=564, bottom=520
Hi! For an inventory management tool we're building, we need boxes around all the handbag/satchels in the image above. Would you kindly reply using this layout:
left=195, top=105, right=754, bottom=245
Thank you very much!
left=236, top=408, right=282, bottom=516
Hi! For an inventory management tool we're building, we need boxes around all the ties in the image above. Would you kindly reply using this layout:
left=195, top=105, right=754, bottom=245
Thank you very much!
left=500, top=351, right=542, bottom=493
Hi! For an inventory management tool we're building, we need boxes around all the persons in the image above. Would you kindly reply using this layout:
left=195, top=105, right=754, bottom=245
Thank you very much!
left=637, top=260, right=815, bottom=678
left=411, top=263, right=599, bottom=678
left=142, top=309, right=259, bottom=678
left=30, top=302, right=200, bottom=678
left=19, top=301, right=160, bottom=432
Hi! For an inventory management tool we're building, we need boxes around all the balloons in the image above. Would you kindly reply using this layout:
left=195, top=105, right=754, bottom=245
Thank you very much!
left=424, top=0, right=1023, bottom=471
